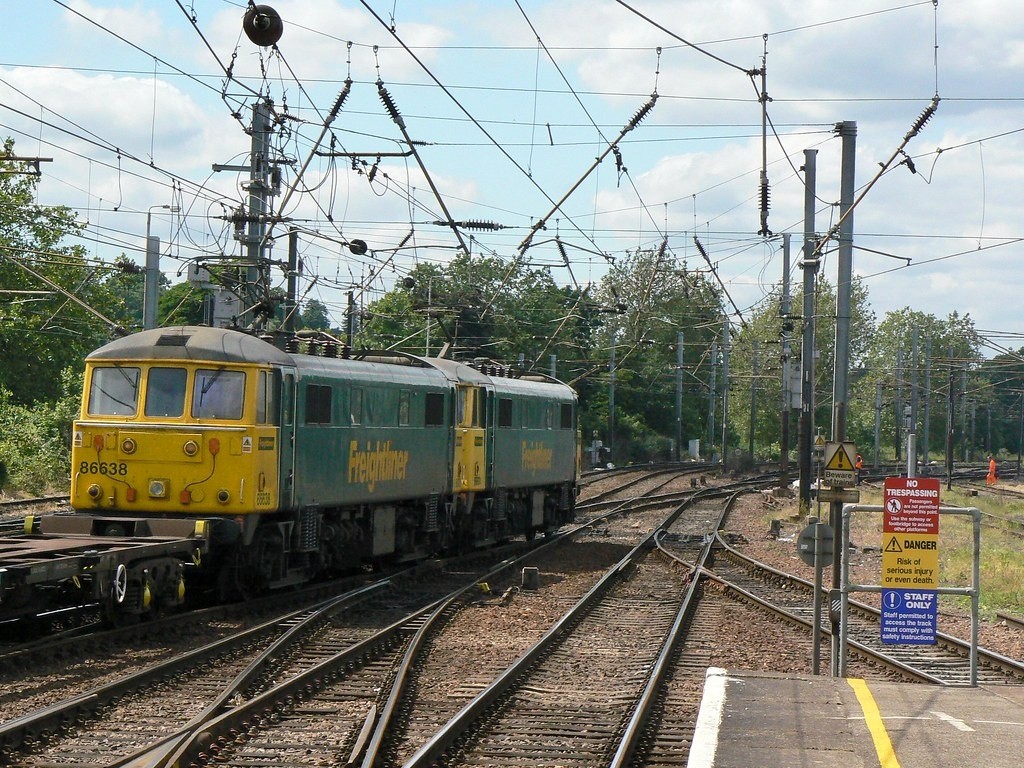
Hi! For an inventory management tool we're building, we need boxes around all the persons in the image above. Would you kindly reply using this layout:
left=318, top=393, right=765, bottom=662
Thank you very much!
left=856, top=452, right=863, bottom=486
left=987, top=456, right=996, bottom=486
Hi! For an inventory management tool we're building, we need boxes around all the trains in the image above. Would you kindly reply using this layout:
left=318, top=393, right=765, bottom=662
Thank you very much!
left=1, top=325, right=582, bottom=634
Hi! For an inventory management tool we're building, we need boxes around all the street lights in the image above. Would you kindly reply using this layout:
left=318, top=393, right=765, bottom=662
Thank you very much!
left=141, top=204, right=183, bottom=331
left=425, top=275, right=455, bottom=356
